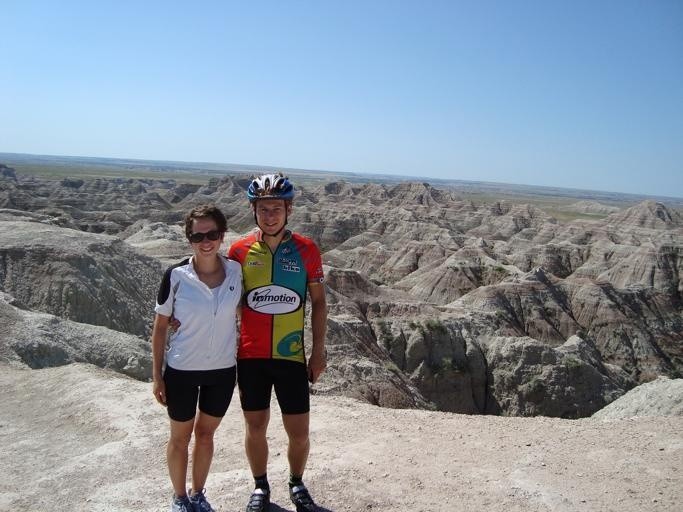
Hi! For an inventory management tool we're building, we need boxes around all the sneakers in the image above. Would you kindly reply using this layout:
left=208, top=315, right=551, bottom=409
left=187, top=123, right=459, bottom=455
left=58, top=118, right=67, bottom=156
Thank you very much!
left=288, top=484, right=317, bottom=511
left=172, top=488, right=215, bottom=511
left=246, top=489, right=271, bottom=512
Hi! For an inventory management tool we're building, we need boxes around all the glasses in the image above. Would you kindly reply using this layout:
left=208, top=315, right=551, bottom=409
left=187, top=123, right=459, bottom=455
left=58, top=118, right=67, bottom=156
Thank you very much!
left=187, top=229, right=222, bottom=243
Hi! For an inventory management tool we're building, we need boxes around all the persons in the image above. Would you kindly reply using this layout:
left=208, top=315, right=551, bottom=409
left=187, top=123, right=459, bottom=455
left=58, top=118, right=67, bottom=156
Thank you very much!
left=148, top=204, right=245, bottom=512
left=167, top=172, right=330, bottom=512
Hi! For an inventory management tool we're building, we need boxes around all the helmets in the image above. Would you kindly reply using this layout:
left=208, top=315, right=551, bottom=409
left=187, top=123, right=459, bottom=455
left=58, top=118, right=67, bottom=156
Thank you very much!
left=247, top=173, right=295, bottom=201
